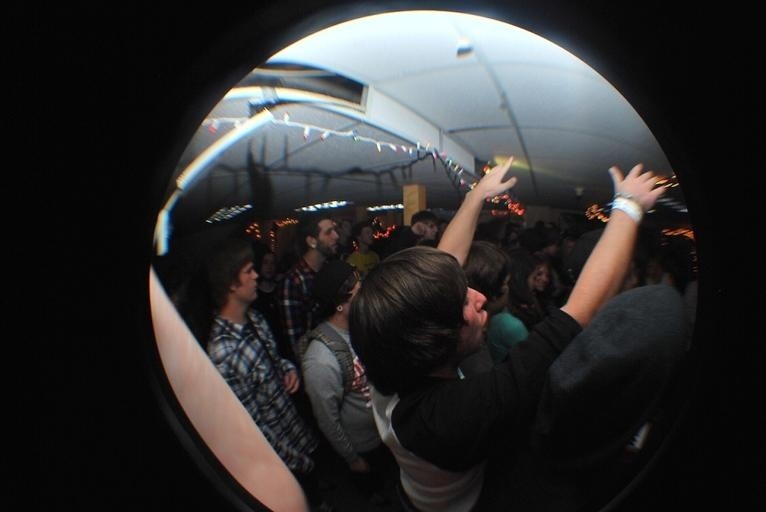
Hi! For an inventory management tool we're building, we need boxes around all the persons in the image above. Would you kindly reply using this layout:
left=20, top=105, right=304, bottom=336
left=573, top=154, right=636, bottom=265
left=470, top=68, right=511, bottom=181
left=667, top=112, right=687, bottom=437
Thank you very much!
left=178, top=154, right=705, bottom=509
